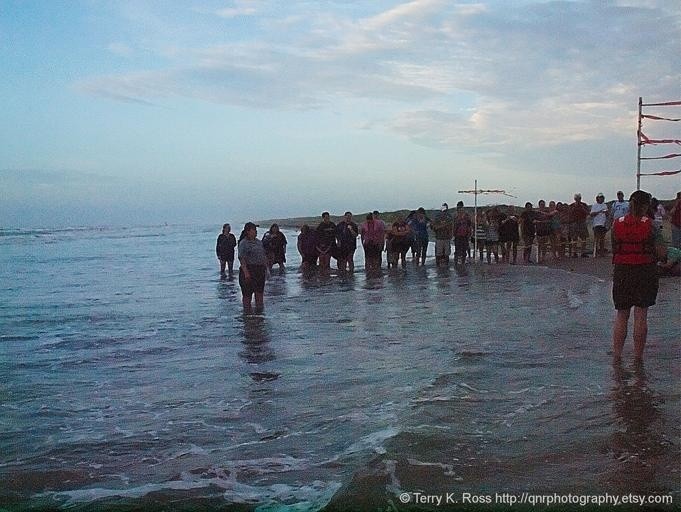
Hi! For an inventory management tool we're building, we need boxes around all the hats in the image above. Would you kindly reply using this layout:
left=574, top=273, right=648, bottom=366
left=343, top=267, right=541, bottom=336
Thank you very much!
left=525, top=201, right=533, bottom=209
left=439, top=201, right=465, bottom=212
left=574, top=190, right=631, bottom=201
left=243, top=221, right=259, bottom=231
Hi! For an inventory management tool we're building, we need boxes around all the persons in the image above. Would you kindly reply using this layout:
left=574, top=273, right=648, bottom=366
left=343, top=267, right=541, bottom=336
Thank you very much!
left=216, top=189, right=680, bottom=278
left=610, top=360, right=659, bottom=482
left=238, top=310, right=278, bottom=382
left=238, top=222, right=266, bottom=310
left=611, top=190, right=666, bottom=360
